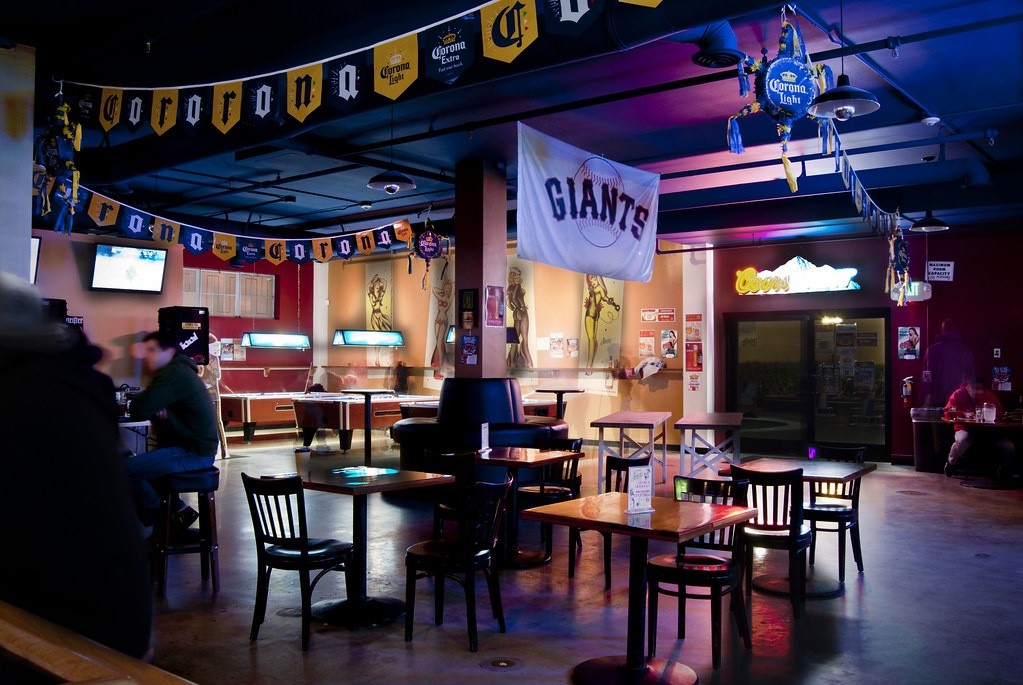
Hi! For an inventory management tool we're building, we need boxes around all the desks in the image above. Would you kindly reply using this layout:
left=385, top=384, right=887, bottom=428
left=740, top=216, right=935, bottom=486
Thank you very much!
left=220, top=392, right=342, bottom=445
left=118, top=420, right=151, bottom=454
left=961, top=420, right=1023, bottom=484
left=291, top=390, right=878, bottom=685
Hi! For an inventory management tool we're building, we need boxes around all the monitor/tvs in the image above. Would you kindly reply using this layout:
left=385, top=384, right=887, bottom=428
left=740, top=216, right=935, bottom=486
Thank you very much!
left=89, top=243, right=169, bottom=295
left=446, top=324, right=455, bottom=343
left=29, top=236, right=41, bottom=285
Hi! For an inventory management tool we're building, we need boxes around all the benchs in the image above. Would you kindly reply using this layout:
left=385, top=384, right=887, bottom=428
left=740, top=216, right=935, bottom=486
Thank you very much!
left=389, top=414, right=569, bottom=504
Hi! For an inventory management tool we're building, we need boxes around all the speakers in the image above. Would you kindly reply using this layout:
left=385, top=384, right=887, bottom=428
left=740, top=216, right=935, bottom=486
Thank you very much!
left=158, top=306, right=209, bottom=365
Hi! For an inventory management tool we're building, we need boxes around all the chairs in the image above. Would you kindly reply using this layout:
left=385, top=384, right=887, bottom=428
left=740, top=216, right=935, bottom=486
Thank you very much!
left=241, top=437, right=866, bottom=670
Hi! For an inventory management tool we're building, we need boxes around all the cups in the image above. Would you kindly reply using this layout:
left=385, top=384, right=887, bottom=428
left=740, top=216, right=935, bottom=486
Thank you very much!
left=984, top=403, right=996, bottom=422
left=976, top=408, right=984, bottom=420
left=949, top=406, right=957, bottom=421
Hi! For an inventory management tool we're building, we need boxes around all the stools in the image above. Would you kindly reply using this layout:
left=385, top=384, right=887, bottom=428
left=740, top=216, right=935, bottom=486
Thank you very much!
left=150, top=466, right=220, bottom=601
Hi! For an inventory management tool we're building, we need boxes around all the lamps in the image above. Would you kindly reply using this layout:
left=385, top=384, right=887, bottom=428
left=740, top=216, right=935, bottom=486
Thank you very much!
left=807, top=0, right=880, bottom=122
left=446, top=324, right=520, bottom=345
left=331, top=249, right=406, bottom=348
left=241, top=262, right=311, bottom=351
left=908, top=209, right=949, bottom=233
left=366, top=101, right=417, bottom=195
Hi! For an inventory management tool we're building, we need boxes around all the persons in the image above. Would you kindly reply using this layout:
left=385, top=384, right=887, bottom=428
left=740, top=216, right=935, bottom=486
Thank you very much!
left=126, top=331, right=218, bottom=542
left=900, top=327, right=920, bottom=359
left=663, top=331, right=677, bottom=358
left=942, top=377, right=1003, bottom=477
left=0, top=286, right=155, bottom=659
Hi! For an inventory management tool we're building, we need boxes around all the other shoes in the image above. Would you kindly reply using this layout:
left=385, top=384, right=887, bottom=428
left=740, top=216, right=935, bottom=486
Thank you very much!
left=175, top=506, right=198, bottom=528
left=944, top=462, right=954, bottom=478
left=146, top=514, right=182, bottom=546
